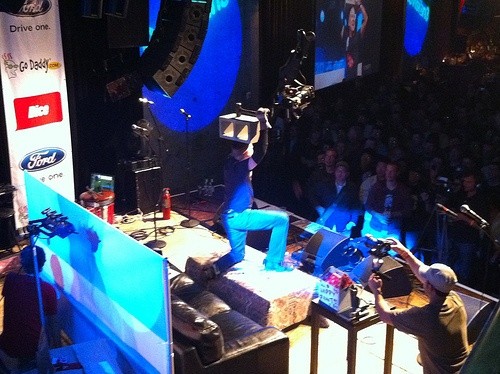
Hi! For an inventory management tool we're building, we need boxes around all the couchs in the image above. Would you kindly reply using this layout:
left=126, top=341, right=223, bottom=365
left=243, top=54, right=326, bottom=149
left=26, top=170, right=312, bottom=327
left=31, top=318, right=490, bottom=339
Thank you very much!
left=167, top=259, right=289, bottom=374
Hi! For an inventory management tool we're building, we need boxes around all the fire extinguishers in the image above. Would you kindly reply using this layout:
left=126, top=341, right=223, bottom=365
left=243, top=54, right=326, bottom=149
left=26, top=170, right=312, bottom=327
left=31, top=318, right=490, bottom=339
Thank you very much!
left=162, top=187, right=171, bottom=220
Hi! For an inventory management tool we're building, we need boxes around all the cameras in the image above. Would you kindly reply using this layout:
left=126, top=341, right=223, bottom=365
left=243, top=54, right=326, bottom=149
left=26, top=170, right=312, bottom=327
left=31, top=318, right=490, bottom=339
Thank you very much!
left=363, top=233, right=393, bottom=260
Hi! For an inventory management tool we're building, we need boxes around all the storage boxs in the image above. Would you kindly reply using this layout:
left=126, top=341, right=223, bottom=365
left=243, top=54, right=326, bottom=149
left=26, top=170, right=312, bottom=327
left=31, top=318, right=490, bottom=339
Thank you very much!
left=85, top=203, right=115, bottom=224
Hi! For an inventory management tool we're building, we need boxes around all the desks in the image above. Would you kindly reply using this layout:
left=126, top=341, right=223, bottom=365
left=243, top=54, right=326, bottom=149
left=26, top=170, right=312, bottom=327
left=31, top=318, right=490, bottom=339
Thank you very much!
left=185, top=246, right=329, bottom=331
left=310, top=286, right=397, bottom=374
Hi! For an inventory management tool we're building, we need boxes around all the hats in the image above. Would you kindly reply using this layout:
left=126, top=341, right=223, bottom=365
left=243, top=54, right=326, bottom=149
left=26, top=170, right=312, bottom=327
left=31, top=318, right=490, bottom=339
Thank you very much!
left=418, top=263, right=458, bottom=293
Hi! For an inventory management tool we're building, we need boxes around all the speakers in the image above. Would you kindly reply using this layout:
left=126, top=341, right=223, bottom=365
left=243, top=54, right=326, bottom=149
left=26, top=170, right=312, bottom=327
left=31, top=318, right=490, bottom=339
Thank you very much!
left=116, top=166, right=163, bottom=214
left=300, top=227, right=350, bottom=274
left=348, top=254, right=411, bottom=298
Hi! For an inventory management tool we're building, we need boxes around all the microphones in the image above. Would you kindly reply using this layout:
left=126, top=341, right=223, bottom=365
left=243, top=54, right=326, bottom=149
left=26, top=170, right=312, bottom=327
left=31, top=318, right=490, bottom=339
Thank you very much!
left=178, top=108, right=191, bottom=120
left=131, top=123, right=148, bottom=132
left=138, top=97, right=155, bottom=105
left=460, top=203, right=491, bottom=229
left=435, top=202, right=460, bottom=219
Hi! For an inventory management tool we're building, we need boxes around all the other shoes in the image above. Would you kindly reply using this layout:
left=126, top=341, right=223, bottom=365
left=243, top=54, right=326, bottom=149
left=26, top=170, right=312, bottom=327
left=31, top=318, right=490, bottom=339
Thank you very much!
left=201, top=264, right=216, bottom=280
left=265, top=263, right=294, bottom=272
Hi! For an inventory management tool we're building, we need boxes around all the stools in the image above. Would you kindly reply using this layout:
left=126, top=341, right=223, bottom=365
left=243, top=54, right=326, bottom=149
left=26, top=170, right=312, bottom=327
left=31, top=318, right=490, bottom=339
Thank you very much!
left=0, top=208, right=20, bottom=253
left=79, top=189, right=115, bottom=218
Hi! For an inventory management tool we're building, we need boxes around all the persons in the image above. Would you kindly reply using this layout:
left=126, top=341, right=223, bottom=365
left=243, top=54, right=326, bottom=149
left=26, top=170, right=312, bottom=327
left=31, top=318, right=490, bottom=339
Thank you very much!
left=340, top=0, right=368, bottom=82
left=0, top=244, right=63, bottom=374
left=367, top=238, right=469, bottom=374
left=252, top=34, right=499, bottom=295
left=201, top=107, right=295, bottom=291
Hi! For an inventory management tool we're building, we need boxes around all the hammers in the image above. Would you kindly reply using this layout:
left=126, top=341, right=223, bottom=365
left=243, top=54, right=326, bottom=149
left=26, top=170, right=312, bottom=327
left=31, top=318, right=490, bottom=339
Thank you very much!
left=236, top=102, right=269, bottom=118
